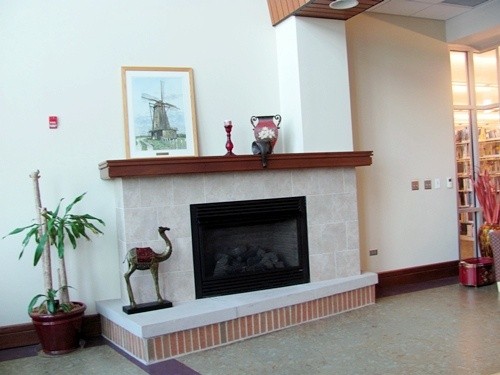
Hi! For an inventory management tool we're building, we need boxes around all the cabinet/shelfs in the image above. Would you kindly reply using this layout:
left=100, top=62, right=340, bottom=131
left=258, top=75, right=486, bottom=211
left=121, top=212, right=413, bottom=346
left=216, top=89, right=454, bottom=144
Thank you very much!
left=455, top=138, right=500, bottom=260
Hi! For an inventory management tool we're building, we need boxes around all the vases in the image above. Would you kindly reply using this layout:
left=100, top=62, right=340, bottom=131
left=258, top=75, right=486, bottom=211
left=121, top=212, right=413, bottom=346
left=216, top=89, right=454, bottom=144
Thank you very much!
left=250, top=114, right=281, bottom=152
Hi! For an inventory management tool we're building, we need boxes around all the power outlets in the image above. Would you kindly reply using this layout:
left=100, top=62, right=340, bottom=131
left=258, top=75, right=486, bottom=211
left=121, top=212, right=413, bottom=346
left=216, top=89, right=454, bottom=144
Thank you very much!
left=435, top=179, right=440, bottom=189
left=424, top=180, right=431, bottom=189
left=411, top=181, right=419, bottom=190
left=370, top=250, right=377, bottom=256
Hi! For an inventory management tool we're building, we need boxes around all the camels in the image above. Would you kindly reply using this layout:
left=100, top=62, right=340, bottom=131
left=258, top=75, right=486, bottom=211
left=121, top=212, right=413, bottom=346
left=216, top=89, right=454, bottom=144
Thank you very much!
left=121, top=227, right=173, bottom=308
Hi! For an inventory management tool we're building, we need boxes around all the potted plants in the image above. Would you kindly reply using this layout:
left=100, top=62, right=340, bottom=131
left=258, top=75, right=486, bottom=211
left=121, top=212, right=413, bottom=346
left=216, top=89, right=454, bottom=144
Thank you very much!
left=1, top=170, right=106, bottom=356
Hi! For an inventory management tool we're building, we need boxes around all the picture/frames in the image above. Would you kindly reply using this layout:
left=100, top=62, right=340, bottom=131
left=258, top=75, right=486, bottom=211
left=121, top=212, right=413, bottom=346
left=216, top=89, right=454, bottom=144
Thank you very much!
left=121, top=66, right=199, bottom=160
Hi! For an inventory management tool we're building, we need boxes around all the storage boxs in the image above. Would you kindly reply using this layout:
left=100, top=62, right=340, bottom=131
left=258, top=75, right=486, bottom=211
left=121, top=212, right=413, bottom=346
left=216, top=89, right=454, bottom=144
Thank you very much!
left=459, top=256, right=495, bottom=287
